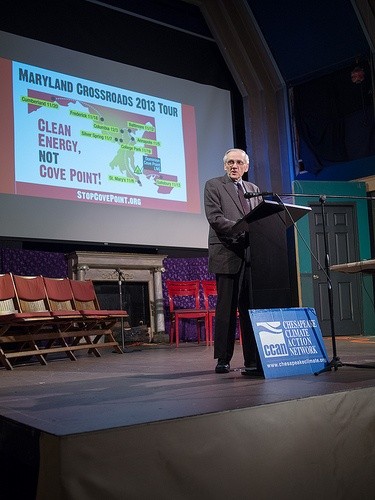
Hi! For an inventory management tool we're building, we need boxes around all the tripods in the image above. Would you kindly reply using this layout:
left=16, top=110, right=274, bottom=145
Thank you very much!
left=271, top=194, right=375, bottom=377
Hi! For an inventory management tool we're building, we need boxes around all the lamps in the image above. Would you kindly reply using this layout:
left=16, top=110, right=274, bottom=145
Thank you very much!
left=350, top=57, right=365, bottom=87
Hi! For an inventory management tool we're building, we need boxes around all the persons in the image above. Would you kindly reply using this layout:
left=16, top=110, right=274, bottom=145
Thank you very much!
left=204, top=149, right=263, bottom=373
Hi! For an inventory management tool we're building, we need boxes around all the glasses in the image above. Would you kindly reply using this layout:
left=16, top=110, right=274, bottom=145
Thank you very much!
left=224, top=161, right=246, bottom=166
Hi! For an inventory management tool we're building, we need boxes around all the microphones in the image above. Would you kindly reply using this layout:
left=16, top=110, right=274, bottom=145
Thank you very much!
left=243, top=191, right=273, bottom=199
left=116, top=267, right=126, bottom=280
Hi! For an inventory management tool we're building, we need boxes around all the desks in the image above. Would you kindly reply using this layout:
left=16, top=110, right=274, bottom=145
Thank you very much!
left=329, top=259, right=375, bottom=312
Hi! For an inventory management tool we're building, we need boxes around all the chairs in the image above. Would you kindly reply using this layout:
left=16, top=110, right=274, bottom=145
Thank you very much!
left=201, top=279, right=243, bottom=346
left=166, top=279, right=209, bottom=347
left=0, top=273, right=129, bottom=371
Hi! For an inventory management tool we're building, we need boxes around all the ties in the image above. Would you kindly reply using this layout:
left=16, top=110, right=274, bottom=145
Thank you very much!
left=237, top=183, right=250, bottom=215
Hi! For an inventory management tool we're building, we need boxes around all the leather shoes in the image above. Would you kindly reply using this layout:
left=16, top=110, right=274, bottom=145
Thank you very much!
left=245, top=361, right=257, bottom=367
left=215, top=362, right=230, bottom=371
